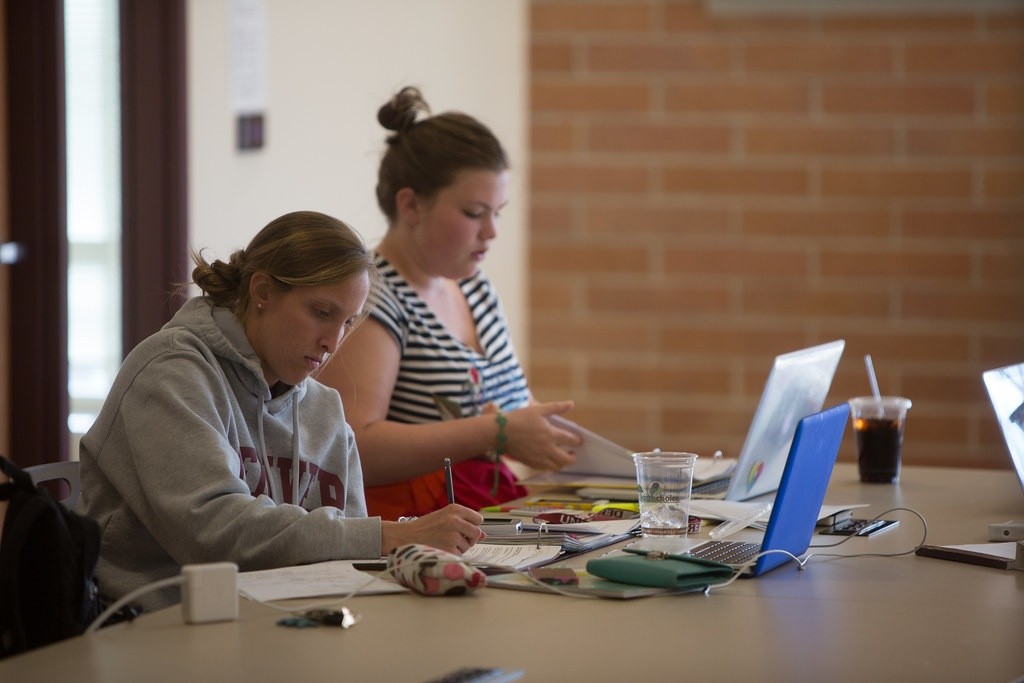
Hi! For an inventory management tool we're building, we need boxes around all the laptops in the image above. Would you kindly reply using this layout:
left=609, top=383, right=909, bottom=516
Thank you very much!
left=578, top=339, right=845, bottom=502
left=596, top=402, right=851, bottom=579
left=980, top=361, right=1024, bottom=491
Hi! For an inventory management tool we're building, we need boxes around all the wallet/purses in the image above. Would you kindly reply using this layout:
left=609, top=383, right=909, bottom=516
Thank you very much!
left=585, top=547, right=734, bottom=587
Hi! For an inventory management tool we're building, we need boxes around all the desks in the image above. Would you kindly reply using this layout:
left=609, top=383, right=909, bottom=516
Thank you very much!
left=0, top=461, right=1024, bottom=683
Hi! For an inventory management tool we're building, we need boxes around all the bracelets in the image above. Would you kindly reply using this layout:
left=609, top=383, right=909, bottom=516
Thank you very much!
left=490, top=411, right=508, bottom=496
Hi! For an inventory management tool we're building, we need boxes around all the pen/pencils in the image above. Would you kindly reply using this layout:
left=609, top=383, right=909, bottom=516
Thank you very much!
left=353, top=562, right=386, bottom=571
left=444, top=457, right=456, bottom=504
left=480, top=506, right=520, bottom=512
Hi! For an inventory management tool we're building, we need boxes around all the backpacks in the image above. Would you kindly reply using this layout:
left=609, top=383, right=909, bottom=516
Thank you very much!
left=0, top=459, right=103, bottom=662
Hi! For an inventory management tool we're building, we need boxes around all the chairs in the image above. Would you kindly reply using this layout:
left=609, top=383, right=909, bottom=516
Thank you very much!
left=17, top=461, right=83, bottom=510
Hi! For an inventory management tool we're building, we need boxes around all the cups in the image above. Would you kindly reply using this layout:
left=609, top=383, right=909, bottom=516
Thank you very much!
left=632, top=451, right=698, bottom=538
left=848, top=395, right=912, bottom=485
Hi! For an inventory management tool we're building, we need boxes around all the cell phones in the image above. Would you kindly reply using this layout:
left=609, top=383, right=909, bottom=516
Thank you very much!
left=477, top=516, right=522, bottom=536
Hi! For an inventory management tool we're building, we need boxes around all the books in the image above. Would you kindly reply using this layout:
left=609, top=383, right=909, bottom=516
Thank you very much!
left=396, top=415, right=736, bottom=571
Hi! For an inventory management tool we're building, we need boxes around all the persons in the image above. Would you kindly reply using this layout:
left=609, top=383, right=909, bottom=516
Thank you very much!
left=311, top=86, right=582, bottom=521
left=80, top=210, right=487, bottom=616
